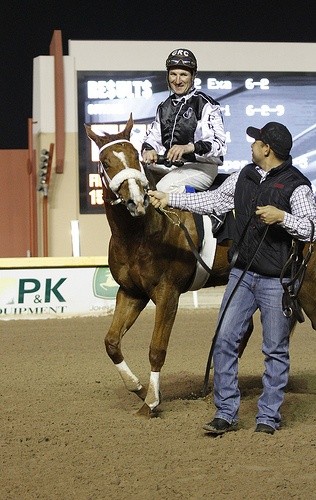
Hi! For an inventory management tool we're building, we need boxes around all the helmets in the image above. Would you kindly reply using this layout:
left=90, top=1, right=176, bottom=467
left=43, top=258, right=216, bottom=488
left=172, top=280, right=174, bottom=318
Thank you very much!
left=165, top=48, right=198, bottom=73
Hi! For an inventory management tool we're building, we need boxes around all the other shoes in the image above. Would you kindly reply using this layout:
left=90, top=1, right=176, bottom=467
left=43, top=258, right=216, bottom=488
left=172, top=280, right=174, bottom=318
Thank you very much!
left=209, top=212, right=226, bottom=233
left=255, top=424, right=275, bottom=435
left=203, top=418, right=239, bottom=433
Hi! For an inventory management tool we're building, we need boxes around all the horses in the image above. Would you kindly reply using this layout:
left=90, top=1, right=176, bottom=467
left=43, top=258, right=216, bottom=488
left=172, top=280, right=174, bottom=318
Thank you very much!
left=81, top=113, right=316, bottom=419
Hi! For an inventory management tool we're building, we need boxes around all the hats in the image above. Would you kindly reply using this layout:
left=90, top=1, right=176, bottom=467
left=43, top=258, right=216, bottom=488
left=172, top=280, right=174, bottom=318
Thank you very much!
left=246, top=121, right=292, bottom=156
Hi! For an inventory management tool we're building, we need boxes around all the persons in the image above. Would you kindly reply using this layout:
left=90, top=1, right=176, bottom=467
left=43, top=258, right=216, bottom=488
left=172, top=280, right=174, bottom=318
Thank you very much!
left=147, top=122, right=316, bottom=433
left=141, top=48, right=228, bottom=194
